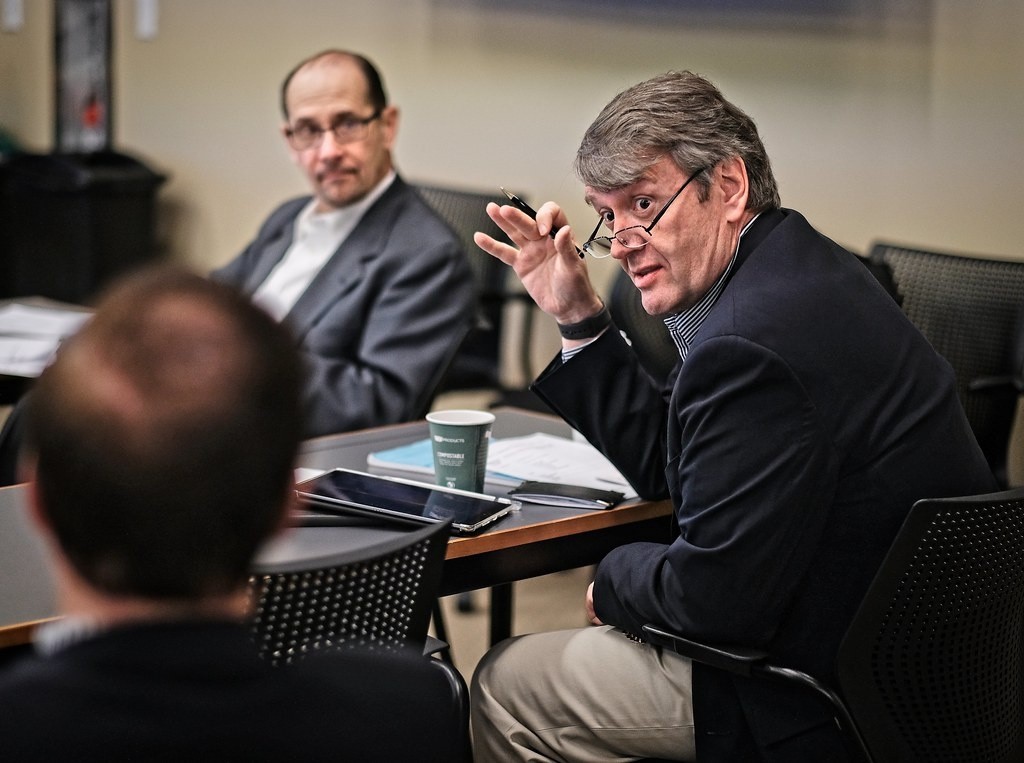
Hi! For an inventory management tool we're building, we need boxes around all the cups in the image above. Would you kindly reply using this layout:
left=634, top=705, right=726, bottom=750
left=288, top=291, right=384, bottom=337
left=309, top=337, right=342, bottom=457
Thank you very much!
left=425, top=411, right=495, bottom=495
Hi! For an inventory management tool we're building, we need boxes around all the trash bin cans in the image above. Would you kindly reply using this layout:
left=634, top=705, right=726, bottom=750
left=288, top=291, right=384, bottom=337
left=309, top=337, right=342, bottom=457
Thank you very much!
left=0, top=146, right=170, bottom=300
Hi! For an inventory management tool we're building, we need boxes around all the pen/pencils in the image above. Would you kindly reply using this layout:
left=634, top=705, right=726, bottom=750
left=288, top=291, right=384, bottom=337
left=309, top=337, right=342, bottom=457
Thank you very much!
left=500, top=185, right=586, bottom=259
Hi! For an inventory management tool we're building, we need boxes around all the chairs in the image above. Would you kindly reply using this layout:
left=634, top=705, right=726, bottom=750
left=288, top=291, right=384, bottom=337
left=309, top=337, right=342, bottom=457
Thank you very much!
left=639, top=490, right=1023, bottom=763
left=237, top=514, right=455, bottom=666
left=410, top=184, right=540, bottom=396
left=868, top=241, right=1024, bottom=488
left=457, top=263, right=687, bottom=647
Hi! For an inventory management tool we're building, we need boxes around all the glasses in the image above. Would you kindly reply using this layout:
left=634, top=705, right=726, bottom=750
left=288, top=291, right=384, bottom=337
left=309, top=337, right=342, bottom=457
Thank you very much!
left=285, top=115, right=378, bottom=151
left=582, top=160, right=718, bottom=259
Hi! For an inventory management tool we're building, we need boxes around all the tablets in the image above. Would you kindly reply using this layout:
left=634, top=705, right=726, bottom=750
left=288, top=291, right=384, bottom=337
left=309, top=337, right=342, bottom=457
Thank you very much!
left=290, top=467, right=513, bottom=531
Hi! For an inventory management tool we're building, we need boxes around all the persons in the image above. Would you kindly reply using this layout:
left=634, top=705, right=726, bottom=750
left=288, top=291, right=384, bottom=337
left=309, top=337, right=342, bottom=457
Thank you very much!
left=198, top=45, right=482, bottom=439
left=468, top=66, right=1005, bottom=763
left=0, top=268, right=477, bottom=762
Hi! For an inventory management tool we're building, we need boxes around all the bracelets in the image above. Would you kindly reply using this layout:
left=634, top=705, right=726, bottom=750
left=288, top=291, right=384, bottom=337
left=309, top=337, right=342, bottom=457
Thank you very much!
left=556, top=294, right=613, bottom=341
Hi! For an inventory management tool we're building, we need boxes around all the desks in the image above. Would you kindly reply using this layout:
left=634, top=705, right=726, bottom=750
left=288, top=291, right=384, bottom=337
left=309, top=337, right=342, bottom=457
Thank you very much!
left=0, top=403, right=677, bottom=648
